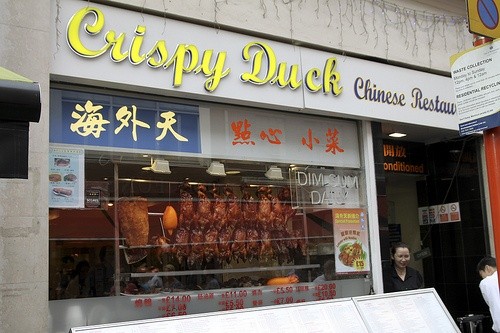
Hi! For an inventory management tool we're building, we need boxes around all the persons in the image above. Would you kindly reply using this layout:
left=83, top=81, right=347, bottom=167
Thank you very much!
left=50, top=245, right=180, bottom=297
left=476, top=256, right=500, bottom=333
left=383, top=242, right=423, bottom=293
left=263, top=255, right=335, bottom=283
